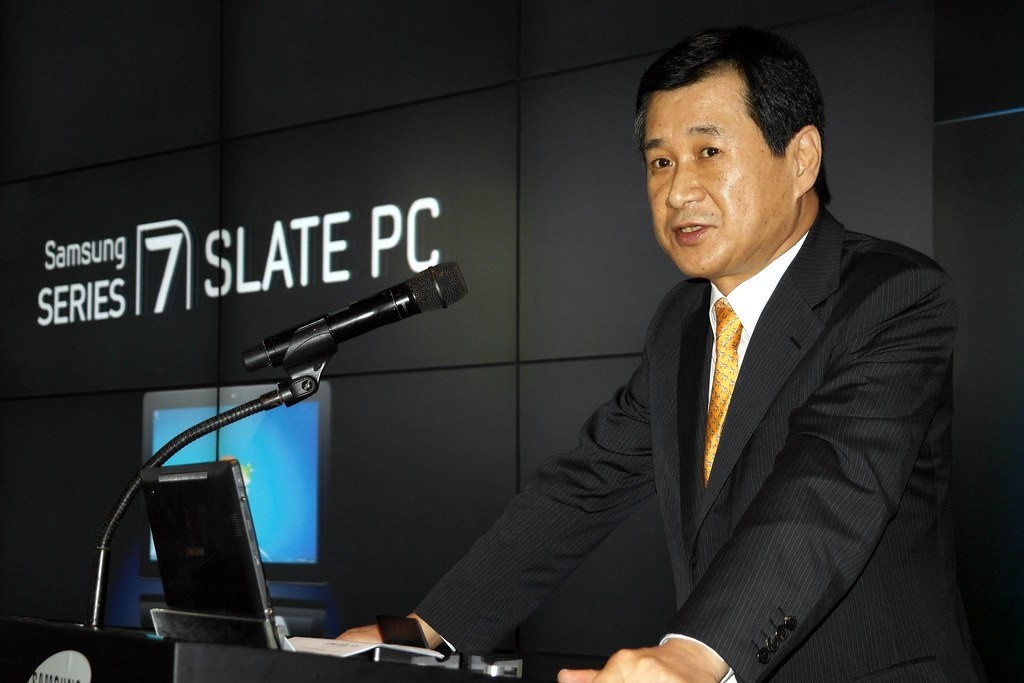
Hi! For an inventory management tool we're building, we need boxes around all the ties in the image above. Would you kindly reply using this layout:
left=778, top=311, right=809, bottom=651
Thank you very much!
left=703, top=296, right=744, bottom=488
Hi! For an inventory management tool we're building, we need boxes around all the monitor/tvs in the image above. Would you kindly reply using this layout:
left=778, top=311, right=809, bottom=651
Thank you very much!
left=141, top=456, right=283, bottom=649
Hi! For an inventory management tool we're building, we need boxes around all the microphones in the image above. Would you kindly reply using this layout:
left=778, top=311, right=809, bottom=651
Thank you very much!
left=241, top=259, right=470, bottom=374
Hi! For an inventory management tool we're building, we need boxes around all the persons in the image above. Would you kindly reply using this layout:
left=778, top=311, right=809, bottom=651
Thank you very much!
left=334, top=26, right=989, bottom=683
